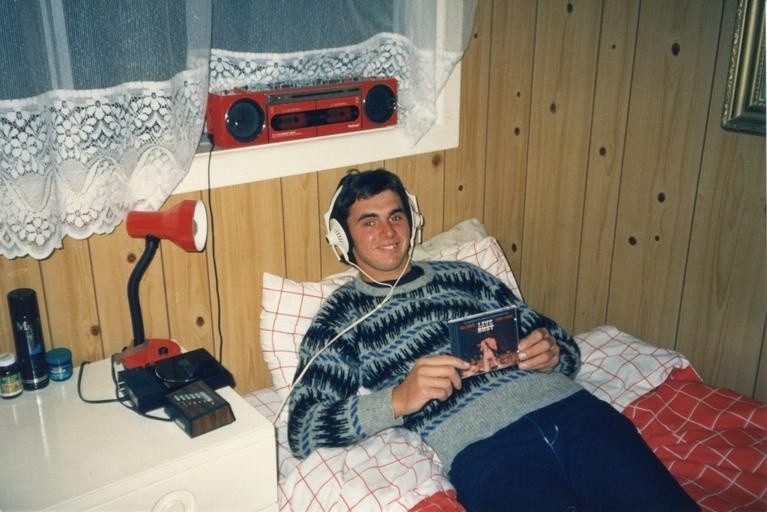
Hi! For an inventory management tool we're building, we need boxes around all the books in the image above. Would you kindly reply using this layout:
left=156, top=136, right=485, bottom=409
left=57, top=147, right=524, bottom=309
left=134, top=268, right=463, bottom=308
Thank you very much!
left=445, top=306, right=520, bottom=378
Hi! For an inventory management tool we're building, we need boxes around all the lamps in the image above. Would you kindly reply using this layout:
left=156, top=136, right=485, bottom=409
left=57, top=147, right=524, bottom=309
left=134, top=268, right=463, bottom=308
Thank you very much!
left=114, top=199, right=209, bottom=370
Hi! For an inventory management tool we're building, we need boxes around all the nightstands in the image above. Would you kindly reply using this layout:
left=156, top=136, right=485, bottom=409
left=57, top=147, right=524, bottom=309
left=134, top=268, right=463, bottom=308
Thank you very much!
left=0, top=338, right=280, bottom=512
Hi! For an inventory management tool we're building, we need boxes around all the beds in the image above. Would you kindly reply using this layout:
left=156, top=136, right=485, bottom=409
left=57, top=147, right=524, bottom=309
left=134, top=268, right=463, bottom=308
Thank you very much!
left=242, top=327, right=767, bottom=512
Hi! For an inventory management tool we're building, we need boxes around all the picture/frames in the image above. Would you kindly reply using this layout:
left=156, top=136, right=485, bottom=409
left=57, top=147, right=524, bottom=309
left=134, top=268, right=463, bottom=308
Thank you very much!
left=721, top=0, right=766, bottom=136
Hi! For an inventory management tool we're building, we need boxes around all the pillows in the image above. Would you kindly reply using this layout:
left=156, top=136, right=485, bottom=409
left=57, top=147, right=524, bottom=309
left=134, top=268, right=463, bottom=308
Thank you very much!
left=320, top=217, right=489, bottom=280
left=259, top=220, right=527, bottom=428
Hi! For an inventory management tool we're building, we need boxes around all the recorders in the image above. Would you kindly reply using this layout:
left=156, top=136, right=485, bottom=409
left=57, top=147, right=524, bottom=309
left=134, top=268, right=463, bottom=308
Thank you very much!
left=208, top=77, right=399, bottom=150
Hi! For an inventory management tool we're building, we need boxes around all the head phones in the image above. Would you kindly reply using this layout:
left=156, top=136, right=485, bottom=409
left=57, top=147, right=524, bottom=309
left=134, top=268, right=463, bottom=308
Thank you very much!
left=321, top=173, right=425, bottom=261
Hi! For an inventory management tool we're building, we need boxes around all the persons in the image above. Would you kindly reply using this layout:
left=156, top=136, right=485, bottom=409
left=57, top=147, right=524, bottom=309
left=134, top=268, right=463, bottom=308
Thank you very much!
left=480, top=331, right=498, bottom=372
left=285, top=167, right=703, bottom=510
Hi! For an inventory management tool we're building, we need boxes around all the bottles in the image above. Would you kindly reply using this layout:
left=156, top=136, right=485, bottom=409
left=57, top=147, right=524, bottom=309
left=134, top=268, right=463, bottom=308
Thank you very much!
left=6, top=288, right=49, bottom=391
